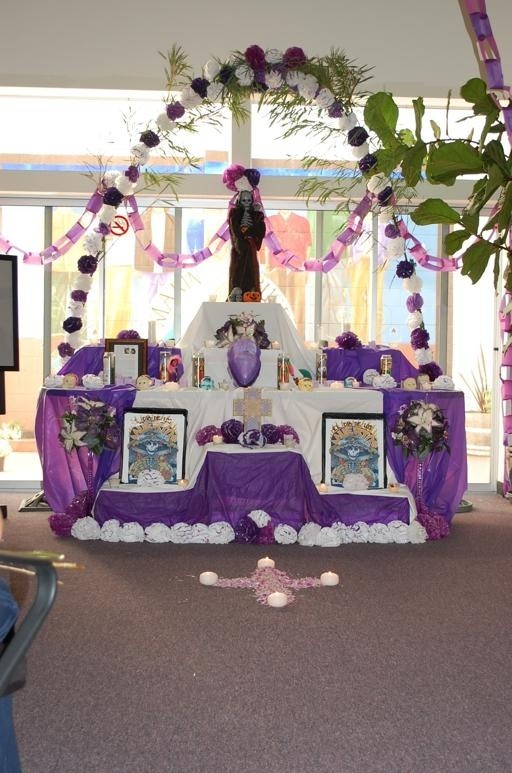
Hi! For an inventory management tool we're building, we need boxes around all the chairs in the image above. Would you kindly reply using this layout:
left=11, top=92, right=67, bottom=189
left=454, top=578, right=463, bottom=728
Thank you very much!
left=0, top=554, right=60, bottom=770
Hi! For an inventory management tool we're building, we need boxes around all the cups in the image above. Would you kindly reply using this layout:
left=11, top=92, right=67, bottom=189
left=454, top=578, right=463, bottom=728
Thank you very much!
left=267, top=295, right=276, bottom=302
left=205, top=339, right=215, bottom=348
left=166, top=340, right=175, bottom=348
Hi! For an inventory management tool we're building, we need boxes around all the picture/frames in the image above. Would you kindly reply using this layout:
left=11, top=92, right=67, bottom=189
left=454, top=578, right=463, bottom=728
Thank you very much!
left=119, top=407, right=188, bottom=485
left=322, top=412, right=387, bottom=489
left=0, top=254, right=19, bottom=371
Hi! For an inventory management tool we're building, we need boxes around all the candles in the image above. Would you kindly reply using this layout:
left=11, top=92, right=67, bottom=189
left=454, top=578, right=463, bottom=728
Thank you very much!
left=198, top=556, right=340, bottom=610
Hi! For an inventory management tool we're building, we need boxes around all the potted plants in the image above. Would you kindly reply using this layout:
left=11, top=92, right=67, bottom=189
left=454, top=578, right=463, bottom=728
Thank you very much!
left=0, top=420, right=22, bottom=472
left=460, top=344, right=493, bottom=457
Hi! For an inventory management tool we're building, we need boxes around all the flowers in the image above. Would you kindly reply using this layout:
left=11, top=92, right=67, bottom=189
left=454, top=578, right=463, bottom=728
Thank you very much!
left=56, top=394, right=118, bottom=456
left=389, top=400, right=453, bottom=461
left=195, top=419, right=300, bottom=451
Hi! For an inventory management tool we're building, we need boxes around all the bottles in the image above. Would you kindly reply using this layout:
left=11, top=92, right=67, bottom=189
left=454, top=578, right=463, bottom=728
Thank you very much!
left=315, top=352, right=328, bottom=385
left=103, top=352, right=116, bottom=386
left=192, top=352, right=205, bottom=388
left=159, top=350, right=171, bottom=384
left=277, top=352, right=289, bottom=390
left=381, top=354, right=392, bottom=377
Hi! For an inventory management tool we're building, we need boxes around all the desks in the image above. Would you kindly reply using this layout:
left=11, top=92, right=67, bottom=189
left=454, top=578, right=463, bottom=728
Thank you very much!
left=40, top=389, right=465, bottom=525
